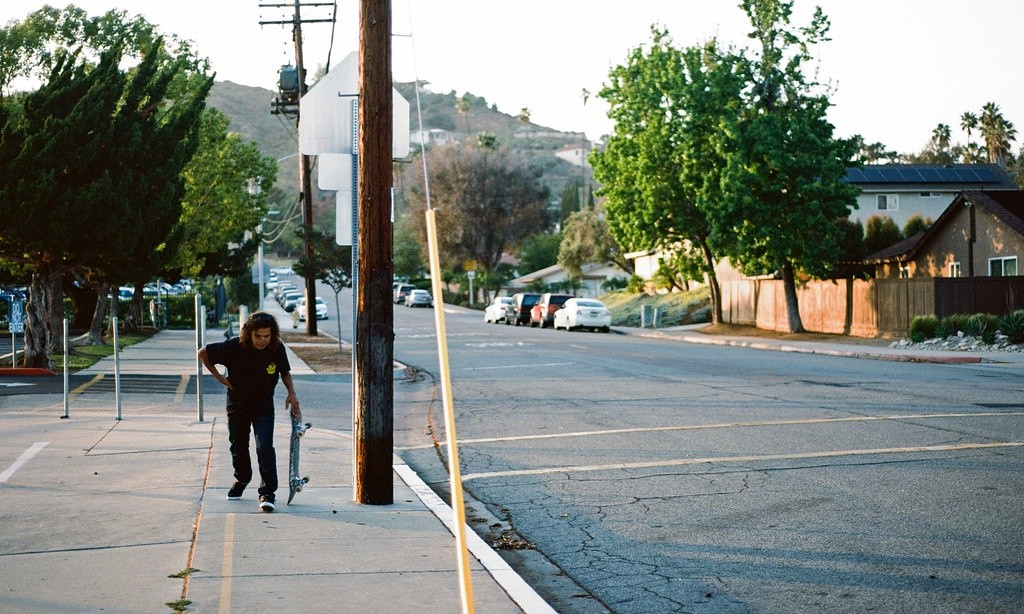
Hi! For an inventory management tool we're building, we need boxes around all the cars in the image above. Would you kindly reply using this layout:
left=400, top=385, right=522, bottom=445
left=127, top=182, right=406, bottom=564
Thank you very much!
left=395, top=284, right=416, bottom=303
left=552, top=297, right=611, bottom=333
left=0, top=279, right=191, bottom=304
left=483, top=296, right=513, bottom=323
left=269, top=266, right=302, bottom=314
left=298, top=296, right=328, bottom=321
left=406, top=289, right=432, bottom=307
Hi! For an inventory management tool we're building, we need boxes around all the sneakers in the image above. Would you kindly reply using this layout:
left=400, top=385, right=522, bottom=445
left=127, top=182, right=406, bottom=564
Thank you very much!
left=258, top=493, right=275, bottom=511
left=228, top=483, right=248, bottom=500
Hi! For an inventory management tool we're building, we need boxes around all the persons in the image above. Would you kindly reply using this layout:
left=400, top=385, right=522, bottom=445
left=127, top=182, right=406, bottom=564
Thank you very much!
left=195, top=311, right=301, bottom=511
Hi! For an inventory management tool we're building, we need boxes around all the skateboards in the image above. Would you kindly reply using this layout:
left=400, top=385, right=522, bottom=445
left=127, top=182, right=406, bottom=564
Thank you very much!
left=287, top=407, right=313, bottom=505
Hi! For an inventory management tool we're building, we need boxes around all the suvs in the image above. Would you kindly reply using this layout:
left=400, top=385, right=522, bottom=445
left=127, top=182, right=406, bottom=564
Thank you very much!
left=528, top=293, right=573, bottom=328
left=504, top=293, right=543, bottom=326
